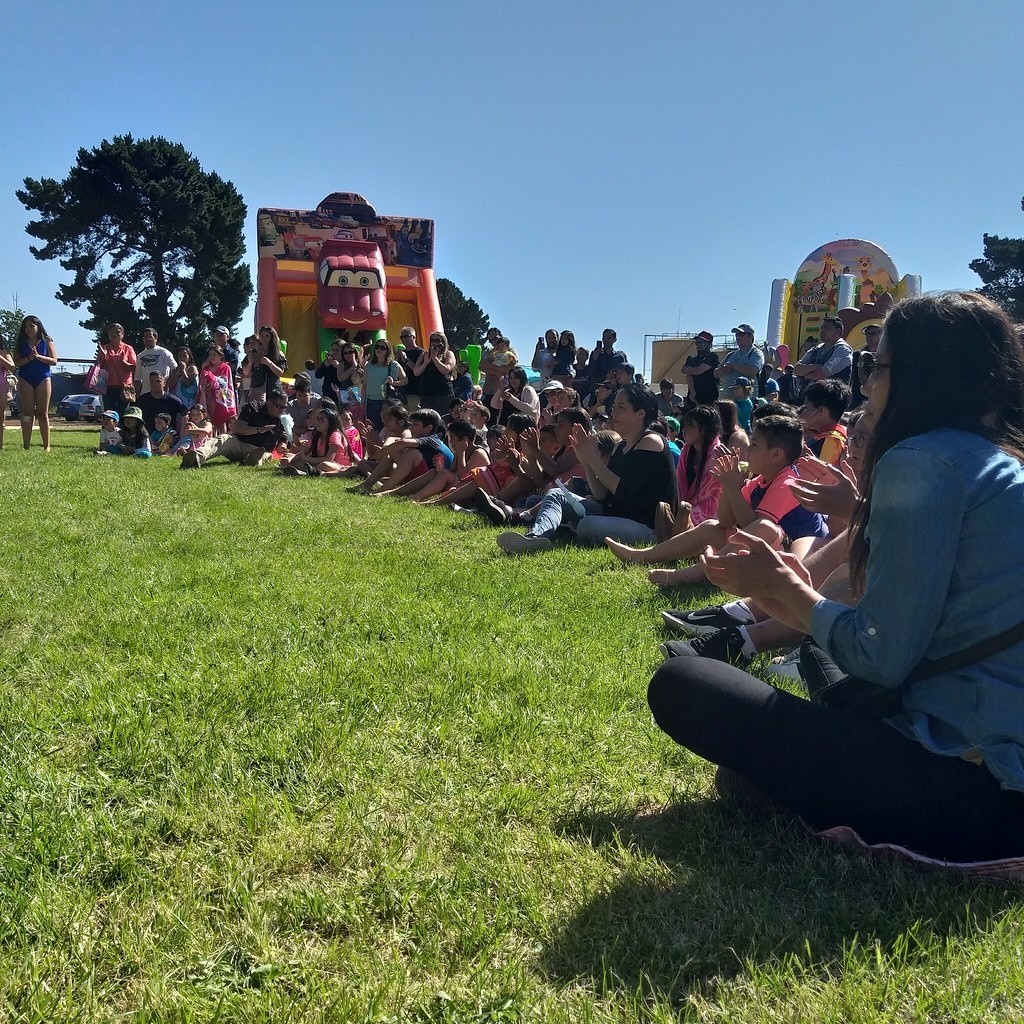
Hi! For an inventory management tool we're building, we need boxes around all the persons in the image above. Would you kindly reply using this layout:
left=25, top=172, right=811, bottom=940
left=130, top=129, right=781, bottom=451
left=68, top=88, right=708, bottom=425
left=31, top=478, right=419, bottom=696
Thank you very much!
left=0, top=315, right=882, bottom=671
left=648, top=290, right=1024, bottom=861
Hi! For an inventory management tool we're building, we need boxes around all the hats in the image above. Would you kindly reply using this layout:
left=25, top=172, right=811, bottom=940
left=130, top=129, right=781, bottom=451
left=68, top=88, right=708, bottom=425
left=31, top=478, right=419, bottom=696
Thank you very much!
left=539, top=362, right=634, bottom=408
left=100, top=406, right=145, bottom=424
left=213, top=326, right=230, bottom=335
left=727, top=363, right=795, bottom=390
left=693, top=324, right=755, bottom=340
left=293, top=372, right=312, bottom=382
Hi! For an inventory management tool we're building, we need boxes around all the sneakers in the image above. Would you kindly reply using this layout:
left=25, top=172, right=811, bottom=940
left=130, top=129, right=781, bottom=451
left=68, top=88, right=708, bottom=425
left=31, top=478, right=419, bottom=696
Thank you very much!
left=662, top=602, right=754, bottom=639
left=660, top=624, right=756, bottom=672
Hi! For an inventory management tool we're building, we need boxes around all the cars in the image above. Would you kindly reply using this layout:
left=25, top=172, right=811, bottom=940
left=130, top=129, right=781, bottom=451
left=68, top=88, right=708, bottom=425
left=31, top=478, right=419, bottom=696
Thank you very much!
left=79, top=394, right=104, bottom=424
left=55, top=394, right=92, bottom=421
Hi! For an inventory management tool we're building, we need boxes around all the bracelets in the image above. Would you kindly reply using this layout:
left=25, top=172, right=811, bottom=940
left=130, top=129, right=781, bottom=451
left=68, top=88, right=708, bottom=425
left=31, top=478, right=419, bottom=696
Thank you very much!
left=27, top=356, right=32, bottom=362
left=405, top=359, right=410, bottom=363
left=256, top=426, right=261, bottom=434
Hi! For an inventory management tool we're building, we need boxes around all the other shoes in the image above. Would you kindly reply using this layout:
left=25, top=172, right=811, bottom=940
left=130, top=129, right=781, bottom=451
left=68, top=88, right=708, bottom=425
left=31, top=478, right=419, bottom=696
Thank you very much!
left=473, top=484, right=572, bottom=555
left=713, top=767, right=771, bottom=815
left=180, top=446, right=323, bottom=478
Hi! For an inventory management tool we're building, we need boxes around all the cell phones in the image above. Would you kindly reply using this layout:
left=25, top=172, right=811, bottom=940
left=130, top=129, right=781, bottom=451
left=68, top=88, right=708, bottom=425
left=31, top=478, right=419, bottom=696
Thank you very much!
left=539, top=337, right=544, bottom=344
left=252, top=349, right=258, bottom=353
left=597, top=341, right=602, bottom=349
left=434, top=343, right=444, bottom=350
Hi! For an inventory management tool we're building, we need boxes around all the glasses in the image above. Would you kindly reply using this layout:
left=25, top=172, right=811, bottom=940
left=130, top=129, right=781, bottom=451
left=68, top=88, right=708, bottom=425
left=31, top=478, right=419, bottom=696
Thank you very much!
left=451, top=409, right=462, bottom=415
left=343, top=333, right=444, bottom=355
left=857, top=351, right=890, bottom=385
left=668, top=411, right=684, bottom=417
left=489, top=336, right=498, bottom=343
left=847, top=434, right=870, bottom=448
left=271, top=400, right=289, bottom=410
left=260, top=325, right=272, bottom=330
left=865, top=332, right=880, bottom=337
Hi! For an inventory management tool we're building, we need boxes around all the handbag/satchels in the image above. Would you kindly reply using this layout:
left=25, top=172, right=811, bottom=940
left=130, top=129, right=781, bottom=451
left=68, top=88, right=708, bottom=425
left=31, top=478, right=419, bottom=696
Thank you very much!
left=387, top=383, right=408, bottom=406
left=84, top=366, right=108, bottom=396
left=120, top=384, right=137, bottom=403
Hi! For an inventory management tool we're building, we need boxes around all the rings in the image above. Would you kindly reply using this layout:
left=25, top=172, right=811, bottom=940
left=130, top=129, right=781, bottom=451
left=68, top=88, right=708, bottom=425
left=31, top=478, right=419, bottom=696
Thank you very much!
left=579, top=435, right=583, bottom=438
left=812, top=477, right=818, bottom=483
